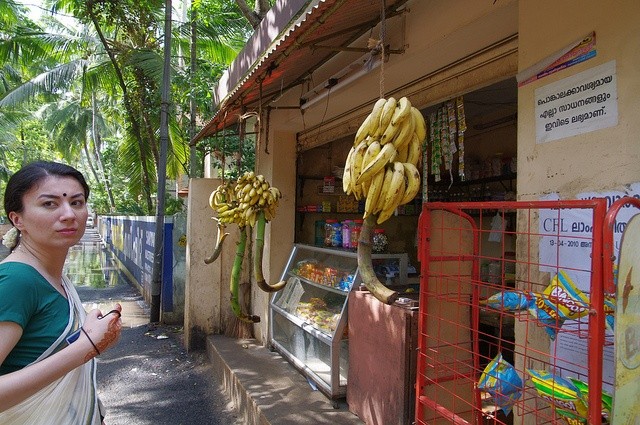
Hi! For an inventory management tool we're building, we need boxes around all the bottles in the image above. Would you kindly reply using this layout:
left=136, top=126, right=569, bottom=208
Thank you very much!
left=331, top=223, right=343, bottom=246
left=371, top=229, right=390, bottom=252
left=324, top=219, right=338, bottom=247
left=342, top=220, right=352, bottom=247
left=351, top=218, right=363, bottom=251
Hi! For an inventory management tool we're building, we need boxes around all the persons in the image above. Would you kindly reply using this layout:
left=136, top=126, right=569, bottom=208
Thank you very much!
left=0, top=159, right=121, bottom=425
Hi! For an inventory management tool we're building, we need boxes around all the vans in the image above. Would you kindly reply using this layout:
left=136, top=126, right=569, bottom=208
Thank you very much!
left=86, top=216, right=94, bottom=228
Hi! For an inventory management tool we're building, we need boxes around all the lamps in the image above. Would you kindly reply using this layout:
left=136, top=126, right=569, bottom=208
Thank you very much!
left=297, top=42, right=389, bottom=110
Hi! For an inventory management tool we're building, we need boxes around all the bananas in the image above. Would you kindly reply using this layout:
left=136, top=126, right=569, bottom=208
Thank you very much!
left=342, top=141, right=421, bottom=225
left=353, top=96, right=427, bottom=165
left=207, top=171, right=282, bottom=229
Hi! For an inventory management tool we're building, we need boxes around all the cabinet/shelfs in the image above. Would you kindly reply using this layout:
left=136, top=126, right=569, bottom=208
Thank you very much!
left=267, top=245, right=408, bottom=409
left=346, top=289, right=470, bottom=424
left=414, top=197, right=640, bottom=425
left=296, top=166, right=516, bottom=215
